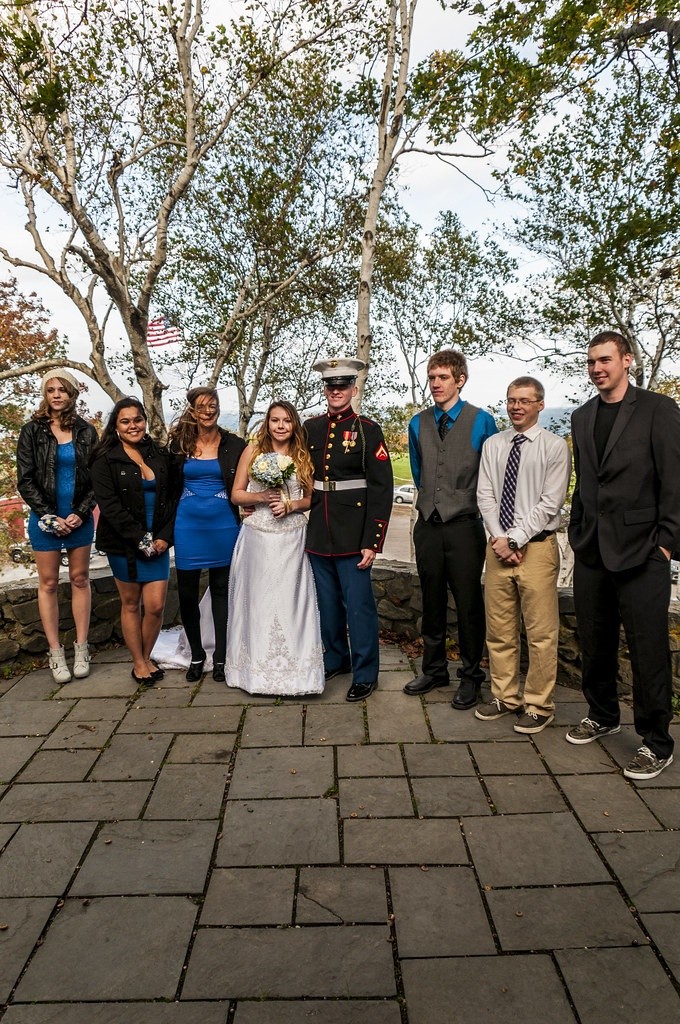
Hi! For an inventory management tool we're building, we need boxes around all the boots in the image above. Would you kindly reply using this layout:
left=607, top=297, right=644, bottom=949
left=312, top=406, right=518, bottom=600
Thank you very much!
left=72, top=640, right=91, bottom=679
left=47, top=643, right=71, bottom=684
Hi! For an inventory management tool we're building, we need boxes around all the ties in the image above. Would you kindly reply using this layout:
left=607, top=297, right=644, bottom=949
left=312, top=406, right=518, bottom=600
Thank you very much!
left=499, top=433, right=528, bottom=533
left=438, top=413, right=450, bottom=441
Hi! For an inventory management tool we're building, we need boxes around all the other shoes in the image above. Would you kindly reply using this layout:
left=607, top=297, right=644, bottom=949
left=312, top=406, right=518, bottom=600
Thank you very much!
left=131, top=658, right=165, bottom=686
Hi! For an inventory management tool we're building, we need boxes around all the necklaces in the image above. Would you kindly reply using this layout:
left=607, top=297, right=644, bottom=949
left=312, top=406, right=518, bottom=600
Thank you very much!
left=138, top=459, right=144, bottom=467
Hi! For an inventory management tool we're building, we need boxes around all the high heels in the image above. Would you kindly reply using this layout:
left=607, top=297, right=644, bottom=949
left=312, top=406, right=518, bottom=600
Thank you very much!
left=212, top=651, right=228, bottom=681
left=186, top=648, right=207, bottom=681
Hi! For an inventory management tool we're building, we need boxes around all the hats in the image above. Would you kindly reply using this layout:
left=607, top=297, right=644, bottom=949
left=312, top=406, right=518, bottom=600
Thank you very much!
left=312, top=357, right=366, bottom=386
left=41, top=368, right=80, bottom=396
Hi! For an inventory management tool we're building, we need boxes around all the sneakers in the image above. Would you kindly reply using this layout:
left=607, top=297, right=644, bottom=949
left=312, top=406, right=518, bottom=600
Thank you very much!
left=565, top=717, right=622, bottom=744
left=514, top=709, right=555, bottom=734
left=624, top=745, right=674, bottom=780
left=474, top=698, right=524, bottom=720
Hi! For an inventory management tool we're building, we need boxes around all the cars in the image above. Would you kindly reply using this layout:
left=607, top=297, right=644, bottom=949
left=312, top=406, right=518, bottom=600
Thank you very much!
left=90, top=530, right=108, bottom=556
left=393, top=484, right=415, bottom=504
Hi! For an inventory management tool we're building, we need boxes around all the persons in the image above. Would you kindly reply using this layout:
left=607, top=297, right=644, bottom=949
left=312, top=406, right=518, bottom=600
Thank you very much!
left=408, top=350, right=500, bottom=710
left=167, top=387, right=246, bottom=681
left=92, top=396, right=176, bottom=686
left=224, top=401, right=326, bottom=697
left=565, top=332, right=680, bottom=780
left=242, top=359, right=394, bottom=702
left=478, top=377, right=571, bottom=734
left=17, top=369, right=99, bottom=684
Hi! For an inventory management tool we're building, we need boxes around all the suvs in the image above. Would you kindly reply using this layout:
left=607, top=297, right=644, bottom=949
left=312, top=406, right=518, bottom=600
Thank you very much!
left=9, top=540, right=70, bottom=567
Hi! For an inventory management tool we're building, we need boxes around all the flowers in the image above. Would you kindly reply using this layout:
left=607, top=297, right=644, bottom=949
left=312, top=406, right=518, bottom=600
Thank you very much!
left=248, top=451, right=298, bottom=490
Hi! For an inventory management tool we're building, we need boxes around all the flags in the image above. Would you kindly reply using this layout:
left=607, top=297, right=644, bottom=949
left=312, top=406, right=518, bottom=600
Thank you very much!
left=147, top=314, right=182, bottom=347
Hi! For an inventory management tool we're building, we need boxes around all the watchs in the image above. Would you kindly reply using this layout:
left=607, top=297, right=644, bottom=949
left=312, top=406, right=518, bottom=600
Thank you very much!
left=507, top=536, right=517, bottom=550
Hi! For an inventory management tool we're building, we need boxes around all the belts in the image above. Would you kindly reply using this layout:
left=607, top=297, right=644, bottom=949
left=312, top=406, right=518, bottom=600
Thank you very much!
left=311, top=479, right=368, bottom=491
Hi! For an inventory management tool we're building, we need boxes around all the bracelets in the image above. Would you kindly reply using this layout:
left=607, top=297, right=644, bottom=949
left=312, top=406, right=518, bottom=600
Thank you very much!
left=287, top=500, right=293, bottom=513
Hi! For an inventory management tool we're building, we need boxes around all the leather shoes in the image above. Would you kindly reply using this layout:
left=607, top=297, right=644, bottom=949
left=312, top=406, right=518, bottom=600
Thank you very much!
left=346, top=679, right=378, bottom=701
left=324, top=667, right=351, bottom=680
left=403, top=671, right=450, bottom=695
left=451, top=678, right=482, bottom=709
